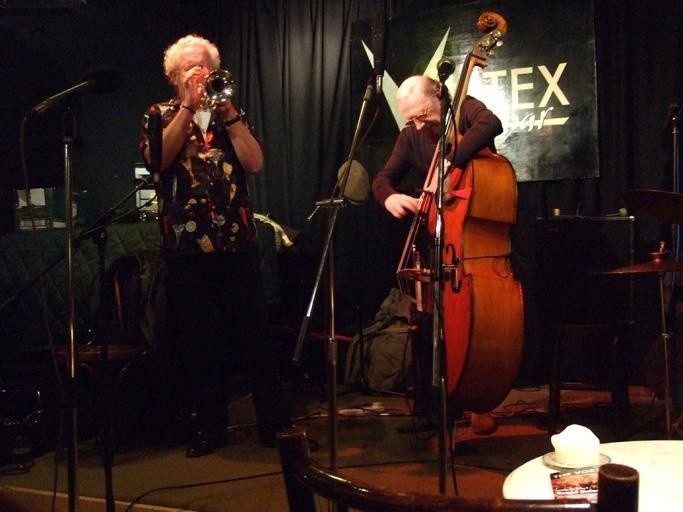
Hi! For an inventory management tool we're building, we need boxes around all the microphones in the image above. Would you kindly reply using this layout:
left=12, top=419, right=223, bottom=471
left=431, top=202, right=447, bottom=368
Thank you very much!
left=438, top=59, right=454, bottom=82
left=149, top=106, right=163, bottom=184
left=372, top=25, right=389, bottom=95
left=32, top=70, right=121, bottom=118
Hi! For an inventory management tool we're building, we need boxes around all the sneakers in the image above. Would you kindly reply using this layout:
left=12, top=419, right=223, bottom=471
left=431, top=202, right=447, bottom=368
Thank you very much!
left=398, top=418, right=453, bottom=432
left=472, top=413, right=496, bottom=434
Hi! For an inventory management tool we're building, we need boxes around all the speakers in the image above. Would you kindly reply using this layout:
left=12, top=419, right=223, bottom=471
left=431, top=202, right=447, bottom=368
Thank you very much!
left=535, top=214, right=641, bottom=324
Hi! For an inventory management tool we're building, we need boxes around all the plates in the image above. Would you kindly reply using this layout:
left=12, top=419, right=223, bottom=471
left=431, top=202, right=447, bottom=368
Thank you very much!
left=540, top=450, right=613, bottom=471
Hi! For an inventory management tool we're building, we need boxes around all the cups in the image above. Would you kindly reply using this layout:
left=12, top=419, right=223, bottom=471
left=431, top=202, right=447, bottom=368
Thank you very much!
left=597, top=462, right=638, bottom=512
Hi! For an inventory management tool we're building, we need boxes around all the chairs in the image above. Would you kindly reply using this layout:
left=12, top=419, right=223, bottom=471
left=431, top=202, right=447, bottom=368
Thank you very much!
left=51, top=257, right=160, bottom=463
left=275, top=423, right=529, bottom=509
left=527, top=212, right=637, bottom=444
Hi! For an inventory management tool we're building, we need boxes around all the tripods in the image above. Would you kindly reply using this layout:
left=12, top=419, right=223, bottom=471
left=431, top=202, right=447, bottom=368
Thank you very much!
left=317, top=210, right=417, bottom=405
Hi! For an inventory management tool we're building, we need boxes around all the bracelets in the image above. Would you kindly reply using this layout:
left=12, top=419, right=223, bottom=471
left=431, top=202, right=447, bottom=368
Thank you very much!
left=223, top=113, right=243, bottom=128
left=180, top=105, right=196, bottom=113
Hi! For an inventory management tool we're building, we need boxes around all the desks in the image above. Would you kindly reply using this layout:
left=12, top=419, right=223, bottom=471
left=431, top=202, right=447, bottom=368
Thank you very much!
left=500, top=437, right=683, bottom=509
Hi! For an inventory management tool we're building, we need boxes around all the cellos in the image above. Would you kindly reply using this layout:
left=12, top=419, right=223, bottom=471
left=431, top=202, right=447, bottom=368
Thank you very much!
left=423, top=12, right=526, bottom=413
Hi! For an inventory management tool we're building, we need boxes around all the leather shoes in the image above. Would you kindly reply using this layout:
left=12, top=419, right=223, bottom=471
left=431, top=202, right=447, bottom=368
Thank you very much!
left=185, top=429, right=227, bottom=457
left=258, top=429, right=320, bottom=452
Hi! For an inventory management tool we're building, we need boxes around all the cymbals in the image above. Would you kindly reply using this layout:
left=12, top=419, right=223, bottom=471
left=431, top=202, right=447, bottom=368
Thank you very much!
left=623, top=190, right=683, bottom=224
left=595, top=261, right=683, bottom=274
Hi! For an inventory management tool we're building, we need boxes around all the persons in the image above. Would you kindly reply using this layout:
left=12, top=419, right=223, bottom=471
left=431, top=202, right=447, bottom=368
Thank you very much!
left=138, top=35, right=292, bottom=458
left=371, top=75, right=502, bottom=434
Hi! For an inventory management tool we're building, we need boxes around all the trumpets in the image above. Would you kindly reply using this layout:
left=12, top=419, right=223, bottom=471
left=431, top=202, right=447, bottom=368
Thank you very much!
left=197, top=69, right=235, bottom=109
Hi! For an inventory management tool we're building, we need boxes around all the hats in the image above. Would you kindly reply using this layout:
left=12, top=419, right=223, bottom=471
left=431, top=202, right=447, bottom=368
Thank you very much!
left=338, top=160, right=371, bottom=206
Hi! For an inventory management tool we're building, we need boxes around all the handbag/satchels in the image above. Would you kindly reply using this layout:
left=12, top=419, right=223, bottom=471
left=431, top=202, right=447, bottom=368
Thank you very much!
left=357, top=289, right=417, bottom=391
